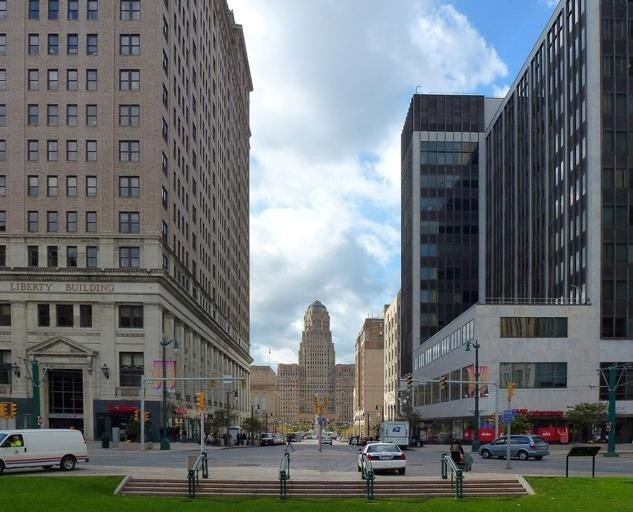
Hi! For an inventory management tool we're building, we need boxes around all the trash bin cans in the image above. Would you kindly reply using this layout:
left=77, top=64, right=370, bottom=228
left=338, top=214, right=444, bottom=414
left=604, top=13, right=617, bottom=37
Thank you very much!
left=102, top=433, right=109, bottom=448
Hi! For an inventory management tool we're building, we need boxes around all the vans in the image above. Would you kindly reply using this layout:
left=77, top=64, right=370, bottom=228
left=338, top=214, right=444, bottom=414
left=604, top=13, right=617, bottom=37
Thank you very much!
left=0, top=428, right=88, bottom=473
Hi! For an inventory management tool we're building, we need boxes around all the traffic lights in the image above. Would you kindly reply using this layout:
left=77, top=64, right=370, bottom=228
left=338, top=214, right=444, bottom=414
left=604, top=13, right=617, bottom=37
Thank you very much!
left=144, top=411, right=149, bottom=422
left=507, top=382, right=515, bottom=398
left=196, top=391, right=204, bottom=411
left=407, top=376, right=412, bottom=388
left=440, top=376, right=446, bottom=391
left=0, top=402, right=17, bottom=417
left=134, top=410, right=138, bottom=421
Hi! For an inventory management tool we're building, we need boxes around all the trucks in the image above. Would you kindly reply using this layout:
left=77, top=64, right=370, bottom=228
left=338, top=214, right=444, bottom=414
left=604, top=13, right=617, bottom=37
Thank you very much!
left=378, top=421, right=409, bottom=450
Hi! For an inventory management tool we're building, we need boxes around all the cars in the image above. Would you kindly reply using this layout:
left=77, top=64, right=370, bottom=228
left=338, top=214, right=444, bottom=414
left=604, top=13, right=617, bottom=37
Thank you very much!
left=287, top=431, right=305, bottom=442
left=260, top=434, right=288, bottom=446
left=478, top=434, right=549, bottom=462
left=357, top=443, right=407, bottom=474
left=321, top=431, right=337, bottom=446
left=341, top=435, right=367, bottom=446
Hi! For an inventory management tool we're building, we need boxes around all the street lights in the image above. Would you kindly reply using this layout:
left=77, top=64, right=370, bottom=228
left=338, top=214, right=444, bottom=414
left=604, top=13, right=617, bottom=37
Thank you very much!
left=226, top=391, right=238, bottom=433
left=398, top=391, right=409, bottom=421
left=265, top=412, right=273, bottom=433
left=354, top=404, right=384, bottom=437
left=251, top=404, right=260, bottom=440
left=160, top=335, right=178, bottom=441
left=466, top=340, right=479, bottom=439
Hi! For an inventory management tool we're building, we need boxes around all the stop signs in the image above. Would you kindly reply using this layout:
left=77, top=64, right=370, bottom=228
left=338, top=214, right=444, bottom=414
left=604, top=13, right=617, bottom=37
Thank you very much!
left=37, top=416, right=44, bottom=425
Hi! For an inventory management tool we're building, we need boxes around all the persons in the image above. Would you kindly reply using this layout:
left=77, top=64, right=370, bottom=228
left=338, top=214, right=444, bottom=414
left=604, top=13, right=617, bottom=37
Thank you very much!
left=7, top=434, right=22, bottom=447
left=411, top=433, right=417, bottom=447
left=462, top=448, right=473, bottom=472
left=202, top=430, right=253, bottom=446
left=449, top=438, right=464, bottom=471
left=284, top=434, right=295, bottom=451
